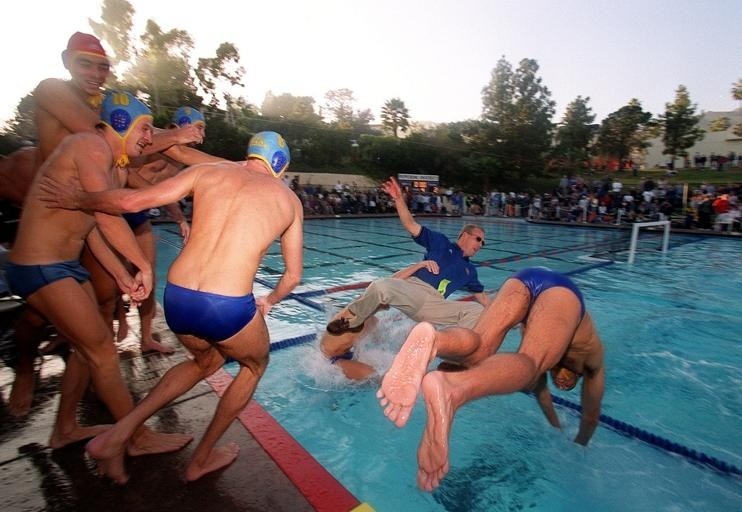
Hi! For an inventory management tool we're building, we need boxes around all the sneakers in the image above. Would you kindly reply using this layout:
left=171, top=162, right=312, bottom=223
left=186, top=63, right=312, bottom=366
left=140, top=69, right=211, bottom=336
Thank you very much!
left=327, top=317, right=364, bottom=332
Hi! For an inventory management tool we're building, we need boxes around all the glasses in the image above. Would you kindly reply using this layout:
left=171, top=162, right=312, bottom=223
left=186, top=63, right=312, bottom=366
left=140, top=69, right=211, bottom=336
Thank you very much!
left=476, top=237, right=485, bottom=246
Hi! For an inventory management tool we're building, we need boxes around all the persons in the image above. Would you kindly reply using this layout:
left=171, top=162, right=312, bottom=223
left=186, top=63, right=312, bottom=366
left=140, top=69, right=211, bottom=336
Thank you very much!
left=694, top=151, right=741, bottom=170
left=0, top=33, right=195, bottom=457
left=376, top=264, right=604, bottom=493
left=39, top=132, right=304, bottom=484
left=482, top=174, right=741, bottom=231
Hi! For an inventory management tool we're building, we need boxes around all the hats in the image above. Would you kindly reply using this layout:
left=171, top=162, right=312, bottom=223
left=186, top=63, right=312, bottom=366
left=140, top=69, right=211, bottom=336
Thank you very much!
left=100, top=89, right=154, bottom=139
left=247, top=131, right=291, bottom=178
left=65, top=32, right=110, bottom=69
left=170, top=106, right=206, bottom=128
